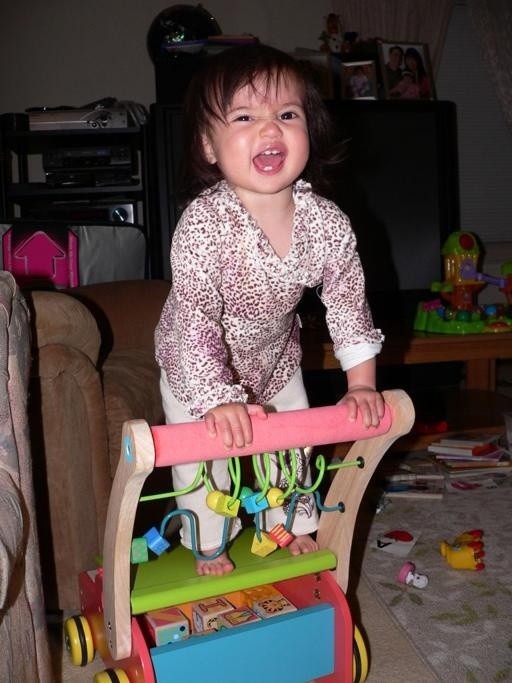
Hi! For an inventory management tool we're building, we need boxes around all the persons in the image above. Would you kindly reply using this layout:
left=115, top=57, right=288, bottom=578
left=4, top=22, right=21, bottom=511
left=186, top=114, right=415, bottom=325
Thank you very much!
left=152, top=40, right=385, bottom=577
left=385, top=46, right=428, bottom=99
left=349, top=65, right=369, bottom=98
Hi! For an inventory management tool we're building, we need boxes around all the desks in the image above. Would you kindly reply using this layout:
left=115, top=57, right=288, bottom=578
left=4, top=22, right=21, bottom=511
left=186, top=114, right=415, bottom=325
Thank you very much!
left=294, top=311, right=510, bottom=475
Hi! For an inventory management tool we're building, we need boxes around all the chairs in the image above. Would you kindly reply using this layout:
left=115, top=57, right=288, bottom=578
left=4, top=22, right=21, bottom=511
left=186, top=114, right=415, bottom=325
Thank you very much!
left=27, top=282, right=179, bottom=620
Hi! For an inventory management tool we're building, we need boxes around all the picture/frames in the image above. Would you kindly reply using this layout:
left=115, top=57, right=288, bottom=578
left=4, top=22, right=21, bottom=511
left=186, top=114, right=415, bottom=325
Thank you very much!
left=289, top=38, right=438, bottom=105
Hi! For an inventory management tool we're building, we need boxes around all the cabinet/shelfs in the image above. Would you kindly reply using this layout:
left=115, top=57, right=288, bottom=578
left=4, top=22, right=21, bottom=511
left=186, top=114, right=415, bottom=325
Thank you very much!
left=0, top=114, right=151, bottom=279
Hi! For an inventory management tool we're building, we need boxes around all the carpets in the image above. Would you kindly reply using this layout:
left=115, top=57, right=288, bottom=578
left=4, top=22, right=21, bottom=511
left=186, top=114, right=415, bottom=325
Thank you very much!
left=351, top=455, right=512, bottom=683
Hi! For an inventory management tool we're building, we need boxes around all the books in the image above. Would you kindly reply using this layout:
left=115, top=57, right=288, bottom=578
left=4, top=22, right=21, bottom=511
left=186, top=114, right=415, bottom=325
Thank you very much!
left=376, top=430, right=511, bottom=499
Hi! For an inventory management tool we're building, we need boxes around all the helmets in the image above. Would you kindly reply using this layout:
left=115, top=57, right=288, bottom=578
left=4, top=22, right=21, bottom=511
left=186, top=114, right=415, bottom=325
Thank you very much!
left=147, top=5, right=222, bottom=70
